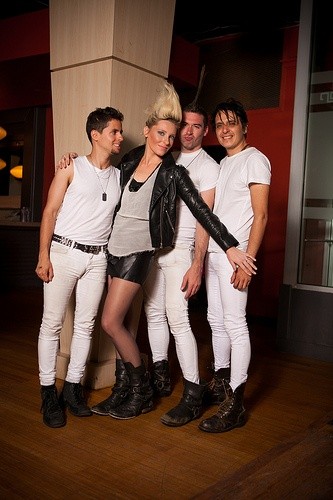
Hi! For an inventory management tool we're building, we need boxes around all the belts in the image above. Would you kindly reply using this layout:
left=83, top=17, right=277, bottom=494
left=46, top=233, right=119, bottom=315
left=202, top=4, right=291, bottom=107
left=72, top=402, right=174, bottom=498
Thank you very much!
left=52, top=233, right=107, bottom=254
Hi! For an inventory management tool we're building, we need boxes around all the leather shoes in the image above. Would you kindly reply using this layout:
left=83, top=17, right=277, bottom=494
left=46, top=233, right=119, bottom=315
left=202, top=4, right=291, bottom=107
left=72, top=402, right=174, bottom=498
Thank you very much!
left=39, top=386, right=65, bottom=427
left=60, top=382, right=90, bottom=416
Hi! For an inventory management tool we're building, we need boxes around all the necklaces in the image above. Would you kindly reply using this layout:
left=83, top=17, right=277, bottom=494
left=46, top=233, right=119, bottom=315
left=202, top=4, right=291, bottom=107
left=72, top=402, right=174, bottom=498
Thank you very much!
left=174, top=150, right=202, bottom=168
left=241, top=144, right=250, bottom=151
left=90, top=154, right=111, bottom=201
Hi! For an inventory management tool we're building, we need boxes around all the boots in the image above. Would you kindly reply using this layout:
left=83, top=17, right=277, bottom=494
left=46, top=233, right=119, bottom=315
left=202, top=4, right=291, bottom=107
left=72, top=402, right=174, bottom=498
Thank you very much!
left=90, top=359, right=131, bottom=415
left=151, top=360, right=172, bottom=396
left=201, top=364, right=231, bottom=403
left=198, top=377, right=247, bottom=433
left=109, top=361, right=153, bottom=419
left=159, top=376, right=207, bottom=427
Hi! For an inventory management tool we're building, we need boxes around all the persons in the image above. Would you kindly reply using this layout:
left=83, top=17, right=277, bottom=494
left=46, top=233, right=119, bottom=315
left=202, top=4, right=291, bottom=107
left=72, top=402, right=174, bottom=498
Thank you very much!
left=198, top=97, right=271, bottom=433
left=142, top=103, right=220, bottom=427
left=35, top=107, right=124, bottom=427
left=54, top=81, right=257, bottom=420
